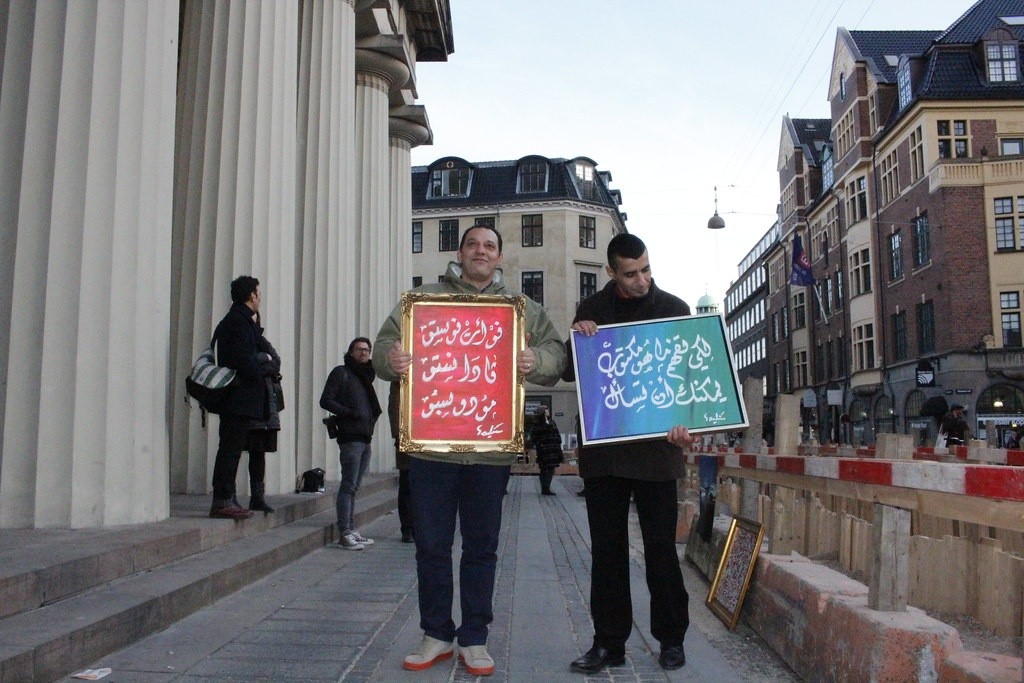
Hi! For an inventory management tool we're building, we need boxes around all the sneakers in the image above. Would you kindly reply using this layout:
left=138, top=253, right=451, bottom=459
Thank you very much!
left=352, top=532, right=374, bottom=545
left=457, top=644, right=494, bottom=676
left=337, top=534, right=365, bottom=550
left=403, top=635, right=454, bottom=670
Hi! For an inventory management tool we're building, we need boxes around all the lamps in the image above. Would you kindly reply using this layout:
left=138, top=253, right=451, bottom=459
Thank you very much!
left=708, top=210, right=726, bottom=229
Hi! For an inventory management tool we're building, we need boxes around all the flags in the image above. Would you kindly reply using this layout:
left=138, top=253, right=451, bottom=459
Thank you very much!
left=790, top=237, right=814, bottom=286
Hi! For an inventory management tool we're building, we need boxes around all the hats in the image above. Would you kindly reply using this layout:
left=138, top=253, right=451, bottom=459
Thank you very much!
left=951, top=404, right=963, bottom=410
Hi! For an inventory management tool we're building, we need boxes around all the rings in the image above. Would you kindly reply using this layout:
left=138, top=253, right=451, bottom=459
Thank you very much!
left=528, top=364, right=530, bottom=368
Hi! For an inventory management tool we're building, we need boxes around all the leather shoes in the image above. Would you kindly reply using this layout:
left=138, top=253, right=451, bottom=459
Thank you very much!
left=570, top=643, right=625, bottom=674
left=660, top=642, right=685, bottom=669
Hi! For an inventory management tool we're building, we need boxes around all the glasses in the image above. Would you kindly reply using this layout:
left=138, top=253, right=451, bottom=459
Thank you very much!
left=351, top=348, right=370, bottom=354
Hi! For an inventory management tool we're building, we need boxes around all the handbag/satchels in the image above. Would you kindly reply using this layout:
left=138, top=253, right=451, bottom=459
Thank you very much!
left=296, top=467, right=326, bottom=494
left=935, top=424, right=949, bottom=448
left=322, top=415, right=339, bottom=440
left=185, top=346, right=241, bottom=413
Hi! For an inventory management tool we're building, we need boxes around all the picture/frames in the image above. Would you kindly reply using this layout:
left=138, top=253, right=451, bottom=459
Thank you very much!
left=705, top=514, right=767, bottom=632
left=399, top=291, right=526, bottom=454
left=569, top=311, right=750, bottom=448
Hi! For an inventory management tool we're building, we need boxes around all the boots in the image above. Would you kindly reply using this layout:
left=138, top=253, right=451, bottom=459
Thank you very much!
left=232, top=483, right=241, bottom=507
left=250, top=481, right=274, bottom=512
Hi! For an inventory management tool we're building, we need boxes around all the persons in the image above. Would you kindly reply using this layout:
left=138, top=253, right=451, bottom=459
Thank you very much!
left=530, top=405, right=562, bottom=496
left=209, top=276, right=281, bottom=518
left=386, top=382, right=415, bottom=543
left=560, top=233, right=700, bottom=674
left=373, top=226, right=570, bottom=676
left=941, top=403, right=968, bottom=445
left=1007, top=427, right=1024, bottom=449
left=319, top=338, right=383, bottom=552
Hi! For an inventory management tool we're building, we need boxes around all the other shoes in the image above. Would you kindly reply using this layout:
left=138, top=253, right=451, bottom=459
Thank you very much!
left=541, top=490, right=556, bottom=495
left=402, top=534, right=414, bottom=542
left=577, top=489, right=585, bottom=496
left=210, top=503, right=253, bottom=518
